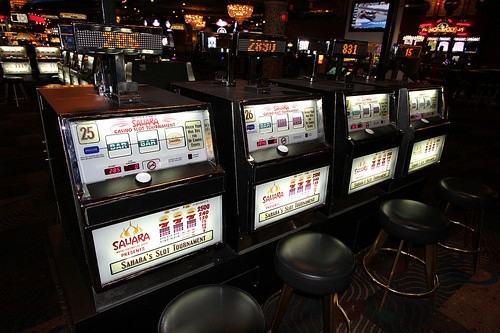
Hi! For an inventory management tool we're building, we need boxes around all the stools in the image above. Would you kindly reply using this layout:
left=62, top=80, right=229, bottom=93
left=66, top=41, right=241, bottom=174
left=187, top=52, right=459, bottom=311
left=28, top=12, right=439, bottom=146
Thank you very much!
left=355, top=198, right=439, bottom=333
left=434, top=176, right=493, bottom=279
left=261, top=232, right=356, bottom=333
left=157, top=284, right=265, bottom=333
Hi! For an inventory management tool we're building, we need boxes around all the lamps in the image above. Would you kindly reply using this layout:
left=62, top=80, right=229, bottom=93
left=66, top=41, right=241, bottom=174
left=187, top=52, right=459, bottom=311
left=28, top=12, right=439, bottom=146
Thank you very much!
left=184, top=14, right=206, bottom=32
left=226, top=4, right=255, bottom=25
